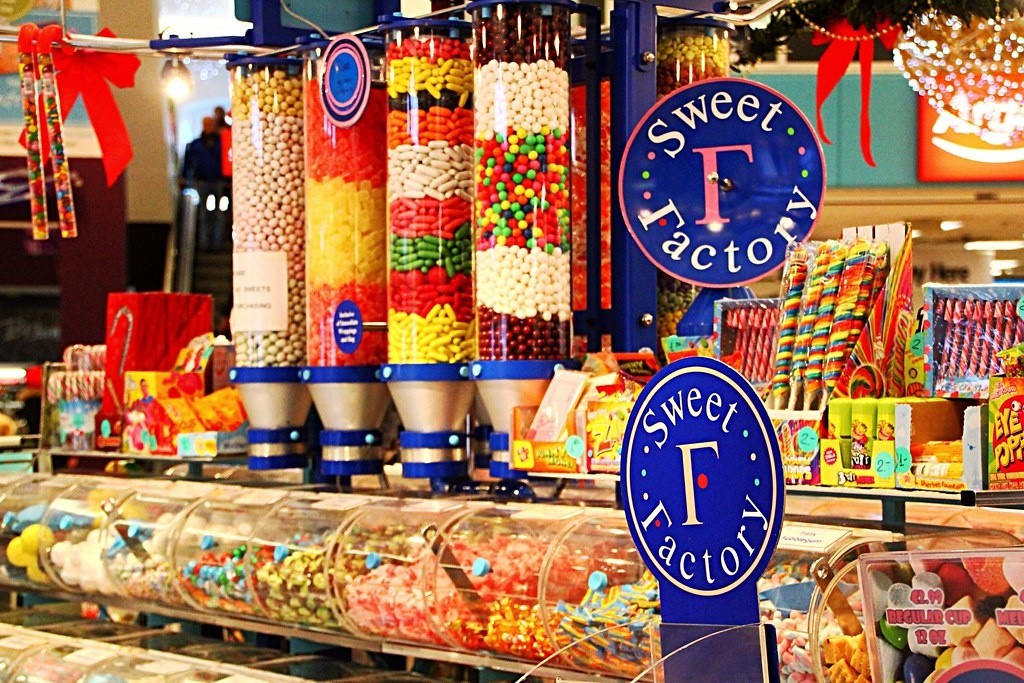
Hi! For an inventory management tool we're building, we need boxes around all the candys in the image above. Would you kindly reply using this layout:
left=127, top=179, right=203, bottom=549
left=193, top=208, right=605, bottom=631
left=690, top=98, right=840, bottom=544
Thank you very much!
left=0, top=4, right=1024, bottom=683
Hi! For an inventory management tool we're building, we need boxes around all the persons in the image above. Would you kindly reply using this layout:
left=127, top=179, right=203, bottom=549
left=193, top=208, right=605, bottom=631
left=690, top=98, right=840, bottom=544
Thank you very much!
left=177, top=116, right=224, bottom=253
left=213, top=107, right=229, bottom=129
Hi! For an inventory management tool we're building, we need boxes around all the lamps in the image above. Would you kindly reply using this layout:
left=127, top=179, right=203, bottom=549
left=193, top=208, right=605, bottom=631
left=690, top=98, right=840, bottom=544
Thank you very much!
left=159, top=49, right=195, bottom=101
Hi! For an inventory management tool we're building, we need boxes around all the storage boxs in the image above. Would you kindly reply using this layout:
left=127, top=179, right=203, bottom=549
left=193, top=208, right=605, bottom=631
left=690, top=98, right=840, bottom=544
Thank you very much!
left=47, top=282, right=1024, bottom=491
